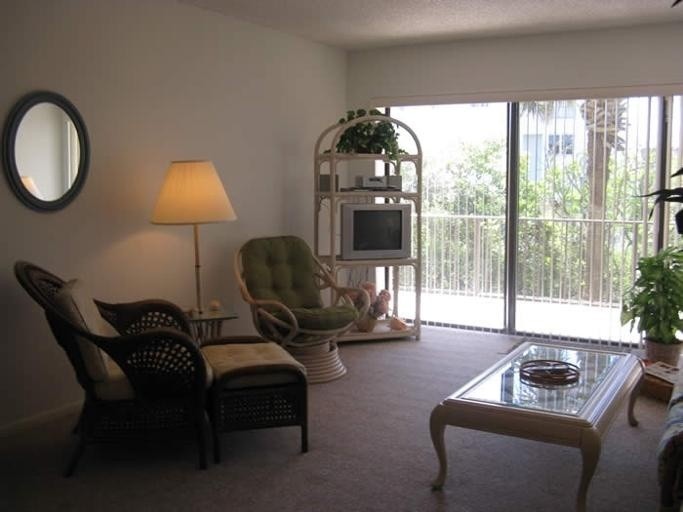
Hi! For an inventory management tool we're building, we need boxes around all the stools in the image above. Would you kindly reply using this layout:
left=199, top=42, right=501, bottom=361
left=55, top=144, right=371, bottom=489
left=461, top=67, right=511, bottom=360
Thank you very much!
left=198, top=335, right=310, bottom=465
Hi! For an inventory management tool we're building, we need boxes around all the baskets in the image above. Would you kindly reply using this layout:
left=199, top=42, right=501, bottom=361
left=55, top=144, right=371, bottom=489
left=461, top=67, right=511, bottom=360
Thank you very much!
left=356, top=317, right=377, bottom=332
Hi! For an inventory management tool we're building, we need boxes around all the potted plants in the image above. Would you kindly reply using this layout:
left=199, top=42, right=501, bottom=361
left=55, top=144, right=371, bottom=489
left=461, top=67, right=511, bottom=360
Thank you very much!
left=323, top=108, right=410, bottom=176
left=620, top=244, right=682, bottom=369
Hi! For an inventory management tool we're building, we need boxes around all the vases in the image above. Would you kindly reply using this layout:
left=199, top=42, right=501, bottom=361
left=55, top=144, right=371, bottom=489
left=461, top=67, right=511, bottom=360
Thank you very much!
left=354, top=318, right=377, bottom=333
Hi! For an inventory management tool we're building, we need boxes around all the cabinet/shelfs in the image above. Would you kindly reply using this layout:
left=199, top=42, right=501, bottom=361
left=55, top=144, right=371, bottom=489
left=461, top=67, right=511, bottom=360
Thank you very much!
left=302, top=117, right=425, bottom=345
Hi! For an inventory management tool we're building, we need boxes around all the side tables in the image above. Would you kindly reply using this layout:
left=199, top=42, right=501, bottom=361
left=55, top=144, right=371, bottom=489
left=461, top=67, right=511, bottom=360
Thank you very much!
left=185, top=304, right=239, bottom=345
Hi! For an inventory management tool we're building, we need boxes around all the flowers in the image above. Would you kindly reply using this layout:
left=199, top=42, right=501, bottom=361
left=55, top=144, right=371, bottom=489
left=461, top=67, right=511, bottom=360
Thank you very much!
left=344, top=281, right=392, bottom=318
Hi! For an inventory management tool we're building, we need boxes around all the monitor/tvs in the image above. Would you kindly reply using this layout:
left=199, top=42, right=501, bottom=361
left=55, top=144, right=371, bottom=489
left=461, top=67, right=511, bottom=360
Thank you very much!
left=341, top=203, right=411, bottom=259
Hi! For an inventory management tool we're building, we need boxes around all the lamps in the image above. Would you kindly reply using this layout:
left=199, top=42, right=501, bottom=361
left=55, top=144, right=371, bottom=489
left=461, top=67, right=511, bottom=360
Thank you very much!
left=19, top=175, right=41, bottom=199
left=152, top=158, right=238, bottom=314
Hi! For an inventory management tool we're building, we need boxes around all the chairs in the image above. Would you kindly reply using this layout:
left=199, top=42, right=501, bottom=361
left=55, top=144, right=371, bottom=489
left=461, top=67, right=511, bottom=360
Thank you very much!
left=232, top=234, right=375, bottom=387
left=12, top=259, right=212, bottom=472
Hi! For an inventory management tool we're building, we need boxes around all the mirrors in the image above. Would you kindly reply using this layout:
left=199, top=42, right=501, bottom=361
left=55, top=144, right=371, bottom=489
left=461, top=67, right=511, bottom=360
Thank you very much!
left=4, top=88, right=91, bottom=214
